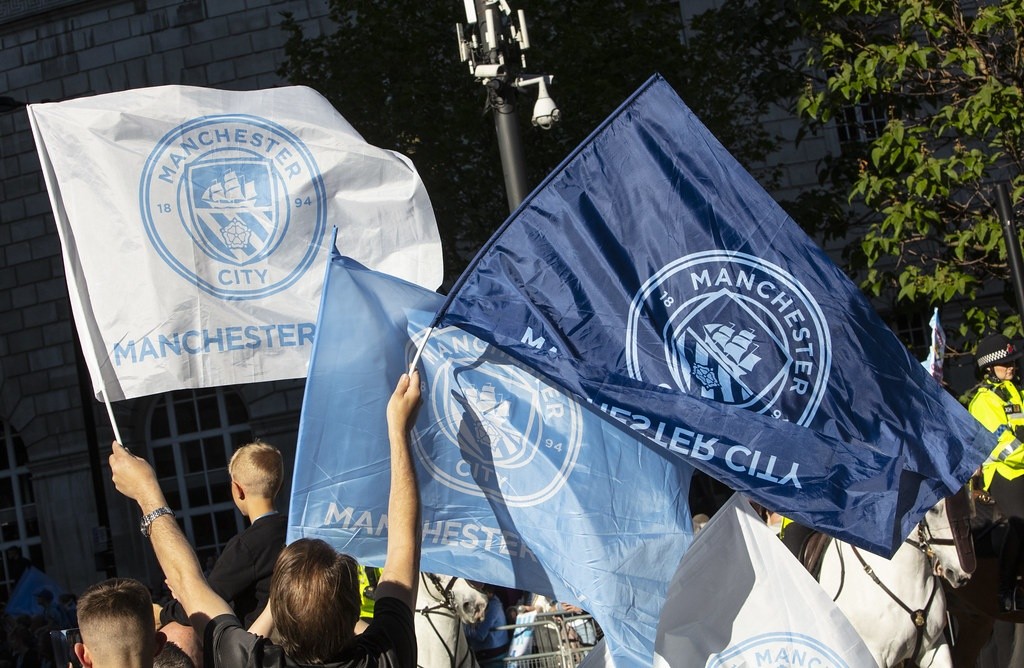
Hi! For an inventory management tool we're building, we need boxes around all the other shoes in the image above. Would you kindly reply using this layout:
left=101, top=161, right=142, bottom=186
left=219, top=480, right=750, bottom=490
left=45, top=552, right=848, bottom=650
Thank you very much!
left=997, top=587, right=1024, bottom=613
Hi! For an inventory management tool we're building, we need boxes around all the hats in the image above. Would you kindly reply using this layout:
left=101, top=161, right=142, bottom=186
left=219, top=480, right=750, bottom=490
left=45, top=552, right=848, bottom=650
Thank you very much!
left=976, top=332, right=1022, bottom=372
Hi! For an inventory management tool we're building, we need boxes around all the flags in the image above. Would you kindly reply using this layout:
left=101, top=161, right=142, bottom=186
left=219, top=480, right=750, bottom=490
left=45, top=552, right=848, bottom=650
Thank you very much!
left=285, top=241, right=692, bottom=668
left=577, top=487, right=877, bottom=668
left=437, top=72, right=1001, bottom=562
left=26, top=83, right=445, bottom=402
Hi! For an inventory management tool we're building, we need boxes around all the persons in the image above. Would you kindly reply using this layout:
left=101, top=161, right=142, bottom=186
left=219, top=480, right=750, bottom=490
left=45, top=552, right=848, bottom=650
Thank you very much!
left=159, top=440, right=289, bottom=631
left=353, top=506, right=828, bottom=668
left=66, top=574, right=200, bottom=668
left=108, top=363, right=425, bottom=668
left=960, top=335, right=1024, bottom=613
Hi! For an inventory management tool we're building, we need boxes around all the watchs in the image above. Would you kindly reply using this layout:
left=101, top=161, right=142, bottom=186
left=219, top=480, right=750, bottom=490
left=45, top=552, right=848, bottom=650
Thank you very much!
left=140, top=507, right=175, bottom=539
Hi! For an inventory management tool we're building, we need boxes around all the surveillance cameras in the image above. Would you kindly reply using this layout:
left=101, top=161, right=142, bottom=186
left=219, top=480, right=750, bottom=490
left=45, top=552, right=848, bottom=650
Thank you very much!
left=475, top=64, right=506, bottom=78
left=531, top=98, right=560, bottom=130
